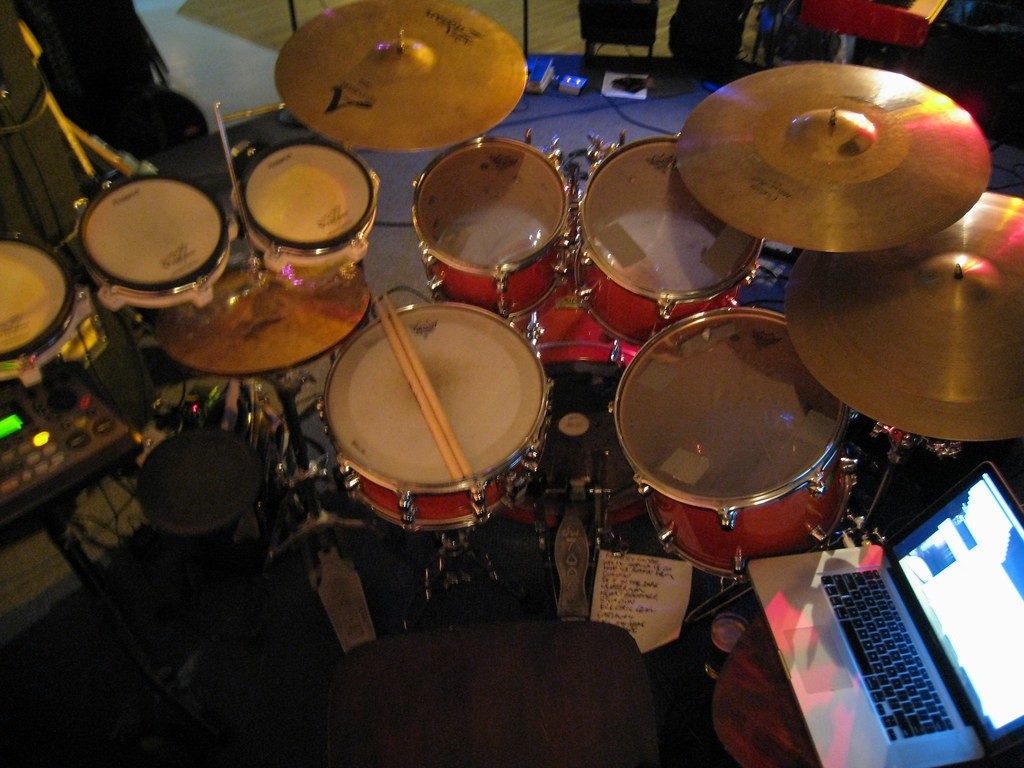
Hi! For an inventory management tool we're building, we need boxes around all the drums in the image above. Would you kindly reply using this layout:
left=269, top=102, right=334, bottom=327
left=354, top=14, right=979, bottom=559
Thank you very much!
left=612, top=306, right=857, bottom=581
left=1, top=228, right=81, bottom=370
left=315, top=302, right=555, bottom=533
left=576, top=131, right=767, bottom=348
left=78, top=177, right=239, bottom=312
left=231, top=139, right=381, bottom=271
left=407, top=136, right=568, bottom=320
left=490, top=278, right=647, bottom=534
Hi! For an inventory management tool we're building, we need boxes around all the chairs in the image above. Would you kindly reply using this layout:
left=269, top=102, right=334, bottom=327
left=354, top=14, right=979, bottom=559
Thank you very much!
left=327, top=621, right=659, bottom=767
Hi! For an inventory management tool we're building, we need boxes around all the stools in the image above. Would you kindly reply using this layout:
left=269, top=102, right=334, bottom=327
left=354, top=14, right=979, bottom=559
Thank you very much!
left=711, top=617, right=821, bottom=768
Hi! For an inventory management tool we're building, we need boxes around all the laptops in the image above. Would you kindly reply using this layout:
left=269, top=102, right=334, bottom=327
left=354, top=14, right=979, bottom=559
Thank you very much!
left=747, top=459, right=1024, bottom=768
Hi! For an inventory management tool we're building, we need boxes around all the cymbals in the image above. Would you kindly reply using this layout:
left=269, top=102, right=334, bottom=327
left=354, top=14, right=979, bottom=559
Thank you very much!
left=152, top=267, right=373, bottom=380
left=675, top=62, right=995, bottom=255
left=783, top=189, right=1024, bottom=442
left=272, top=1, right=531, bottom=155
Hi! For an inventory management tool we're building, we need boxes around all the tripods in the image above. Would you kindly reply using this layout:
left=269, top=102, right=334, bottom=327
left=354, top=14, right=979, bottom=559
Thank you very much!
left=238, top=365, right=630, bottom=652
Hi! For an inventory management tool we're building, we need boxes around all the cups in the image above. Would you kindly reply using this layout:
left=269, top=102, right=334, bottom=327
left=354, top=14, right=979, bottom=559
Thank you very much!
left=704, top=612, right=750, bottom=680
